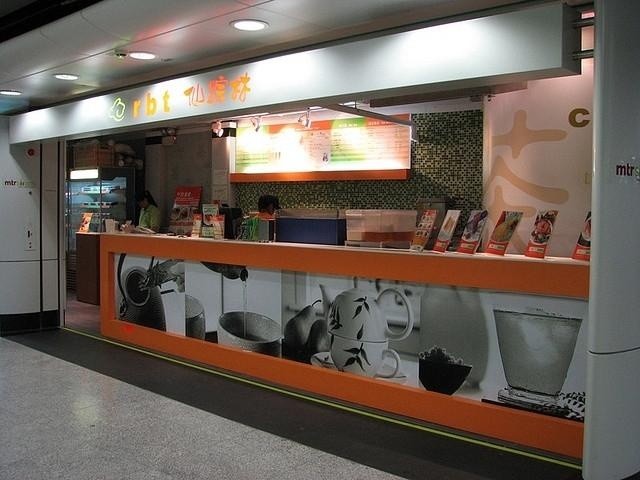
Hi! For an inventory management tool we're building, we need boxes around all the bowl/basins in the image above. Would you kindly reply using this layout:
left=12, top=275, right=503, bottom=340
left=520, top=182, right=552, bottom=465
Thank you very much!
left=419, top=357, right=472, bottom=395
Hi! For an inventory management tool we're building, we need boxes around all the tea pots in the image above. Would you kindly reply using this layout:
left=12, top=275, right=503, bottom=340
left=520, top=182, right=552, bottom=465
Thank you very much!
left=314, top=278, right=414, bottom=343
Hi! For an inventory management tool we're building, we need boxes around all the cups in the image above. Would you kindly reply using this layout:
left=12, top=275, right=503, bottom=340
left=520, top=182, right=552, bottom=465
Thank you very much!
left=217, top=310, right=282, bottom=358
left=327, top=331, right=402, bottom=379
left=184, top=294, right=206, bottom=339
left=494, top=306, right=582, bottom=413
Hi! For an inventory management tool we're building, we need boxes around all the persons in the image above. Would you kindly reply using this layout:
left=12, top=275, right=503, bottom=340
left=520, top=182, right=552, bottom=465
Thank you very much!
left=133, top=188, right=160, bottom=233
left=258, top=194, right=281, bottom=215
